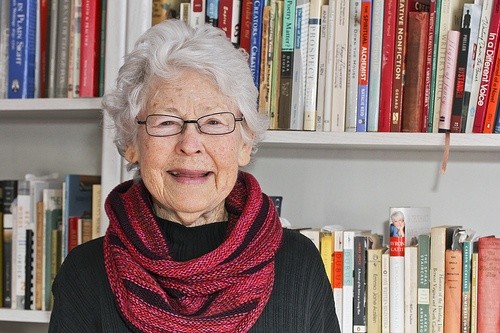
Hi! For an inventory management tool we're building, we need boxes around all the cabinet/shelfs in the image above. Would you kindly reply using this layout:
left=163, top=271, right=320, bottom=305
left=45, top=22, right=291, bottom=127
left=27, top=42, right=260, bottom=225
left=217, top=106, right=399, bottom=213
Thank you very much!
left=0, top=0, right=127, bottom=323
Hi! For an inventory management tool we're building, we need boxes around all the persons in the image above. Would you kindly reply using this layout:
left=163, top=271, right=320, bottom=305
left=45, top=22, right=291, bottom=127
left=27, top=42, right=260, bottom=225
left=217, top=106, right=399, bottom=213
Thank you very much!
left=49, top=16, right=342, bottom=333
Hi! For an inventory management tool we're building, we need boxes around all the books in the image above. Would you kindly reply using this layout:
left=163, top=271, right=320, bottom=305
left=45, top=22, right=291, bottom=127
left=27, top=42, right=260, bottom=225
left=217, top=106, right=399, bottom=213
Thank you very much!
left=0, top=0, right=500, bottom=333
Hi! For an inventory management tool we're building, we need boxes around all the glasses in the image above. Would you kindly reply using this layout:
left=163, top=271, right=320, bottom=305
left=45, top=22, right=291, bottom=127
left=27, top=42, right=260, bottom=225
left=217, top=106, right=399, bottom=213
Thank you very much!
left=137, top=111, right=243, bottom=137
